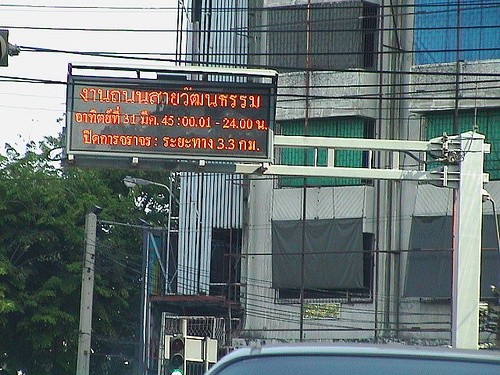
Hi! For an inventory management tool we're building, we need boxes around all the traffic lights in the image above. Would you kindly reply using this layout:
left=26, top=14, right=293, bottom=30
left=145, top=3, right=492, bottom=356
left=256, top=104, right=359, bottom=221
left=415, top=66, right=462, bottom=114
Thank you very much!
left=0, top=28, right=8, bottom=67
left=169, top=335, right=185, bottom=375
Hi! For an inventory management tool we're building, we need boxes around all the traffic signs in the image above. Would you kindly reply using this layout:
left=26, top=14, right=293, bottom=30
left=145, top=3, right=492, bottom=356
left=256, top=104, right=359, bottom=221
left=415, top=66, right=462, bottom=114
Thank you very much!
left=63, top=75, right=279, bottom=164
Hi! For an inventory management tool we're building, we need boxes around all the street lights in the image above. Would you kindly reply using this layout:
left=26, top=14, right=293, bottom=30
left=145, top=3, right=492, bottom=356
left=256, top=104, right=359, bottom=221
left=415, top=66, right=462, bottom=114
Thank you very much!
left=123, top=174, right=180, bottom=208
